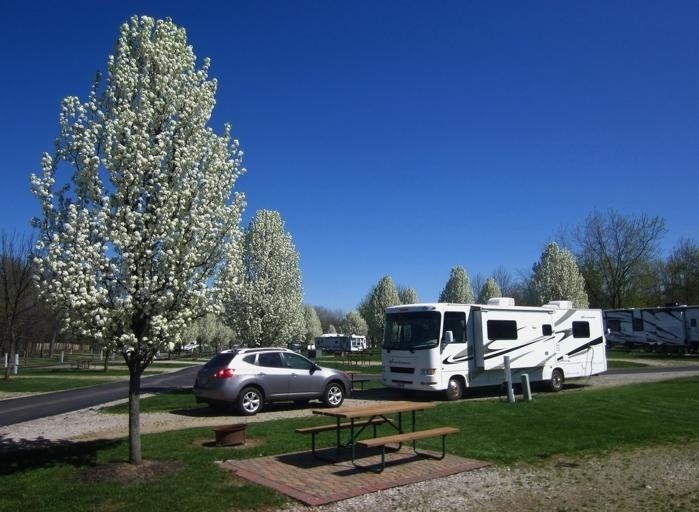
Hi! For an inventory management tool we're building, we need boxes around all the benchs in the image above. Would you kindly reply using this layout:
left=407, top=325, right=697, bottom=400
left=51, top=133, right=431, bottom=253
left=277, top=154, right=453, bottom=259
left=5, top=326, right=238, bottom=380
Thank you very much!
left=295, top=418, right=461, bottom=474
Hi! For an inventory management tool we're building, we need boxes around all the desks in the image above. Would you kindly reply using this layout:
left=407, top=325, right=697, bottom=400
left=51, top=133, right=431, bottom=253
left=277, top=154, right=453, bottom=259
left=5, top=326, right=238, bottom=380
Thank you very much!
left=311, top=400, right=447, bottom=473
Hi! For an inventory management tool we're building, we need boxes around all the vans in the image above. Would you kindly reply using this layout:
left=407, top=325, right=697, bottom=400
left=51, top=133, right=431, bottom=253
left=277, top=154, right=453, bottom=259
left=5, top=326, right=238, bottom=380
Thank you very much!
left=381, top=297, right=608, bottom=401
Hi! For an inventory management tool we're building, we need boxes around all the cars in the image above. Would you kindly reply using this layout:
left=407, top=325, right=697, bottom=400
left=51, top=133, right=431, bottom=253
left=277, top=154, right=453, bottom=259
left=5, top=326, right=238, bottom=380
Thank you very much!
left=194, top=347, right=353, bottom=416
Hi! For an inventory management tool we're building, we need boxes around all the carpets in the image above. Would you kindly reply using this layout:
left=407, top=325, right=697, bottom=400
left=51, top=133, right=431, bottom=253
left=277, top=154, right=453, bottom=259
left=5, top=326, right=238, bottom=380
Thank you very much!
left=214, top=443, right=493, bottom=506
